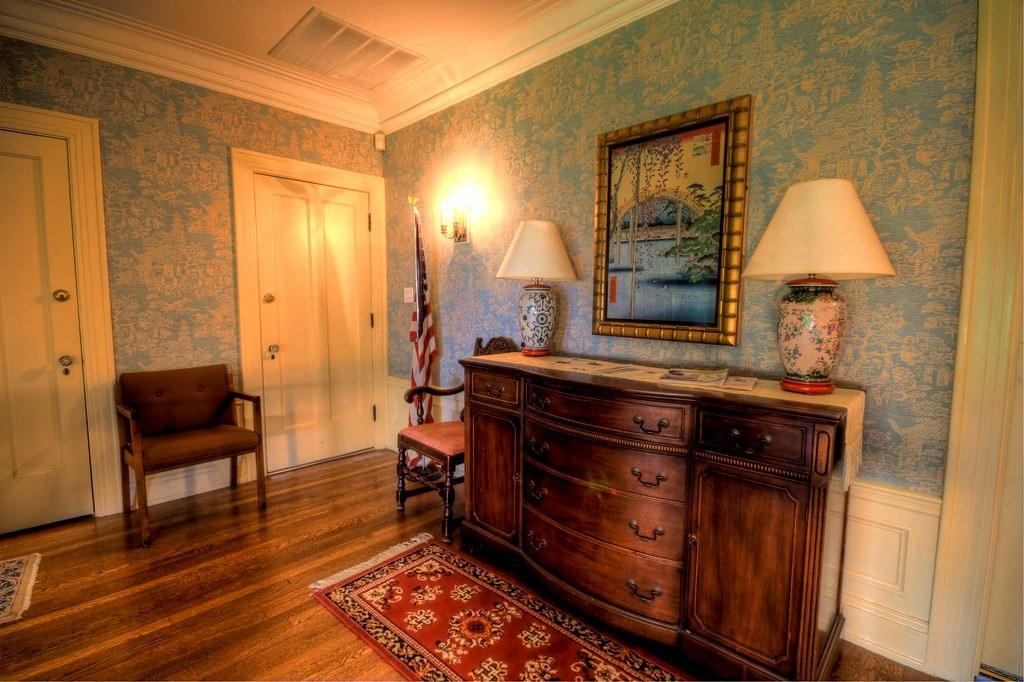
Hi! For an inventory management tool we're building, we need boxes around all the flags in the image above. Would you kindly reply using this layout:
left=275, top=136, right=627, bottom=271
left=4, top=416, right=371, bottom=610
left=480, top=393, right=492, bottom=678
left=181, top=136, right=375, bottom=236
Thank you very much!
left=406, top=211, right=439, bottom=470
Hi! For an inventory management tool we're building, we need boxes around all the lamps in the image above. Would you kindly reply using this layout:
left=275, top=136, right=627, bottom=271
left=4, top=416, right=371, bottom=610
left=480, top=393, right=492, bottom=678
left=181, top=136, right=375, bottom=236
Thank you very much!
left=438, top=200, right=469, bottom=244
left=686, top=178, right=896, bottom=395
left=494, top=219, right=579, bottom=358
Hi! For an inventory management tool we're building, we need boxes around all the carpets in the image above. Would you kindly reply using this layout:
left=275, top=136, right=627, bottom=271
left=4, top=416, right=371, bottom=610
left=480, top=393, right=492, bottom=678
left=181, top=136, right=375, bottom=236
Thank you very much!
left=308, top=531, right=696, bottom=682
left=0, top=553, right=41, bottom=625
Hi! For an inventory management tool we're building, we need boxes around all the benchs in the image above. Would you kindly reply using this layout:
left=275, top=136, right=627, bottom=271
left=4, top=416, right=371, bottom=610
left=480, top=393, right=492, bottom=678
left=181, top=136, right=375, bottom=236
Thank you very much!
left=114, top=364, right=267, bottom=549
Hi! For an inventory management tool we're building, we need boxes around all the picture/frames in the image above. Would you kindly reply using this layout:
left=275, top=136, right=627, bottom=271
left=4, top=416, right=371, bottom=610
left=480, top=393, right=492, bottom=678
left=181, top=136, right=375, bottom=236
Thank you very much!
left=592, top=92, right=757, bottom=347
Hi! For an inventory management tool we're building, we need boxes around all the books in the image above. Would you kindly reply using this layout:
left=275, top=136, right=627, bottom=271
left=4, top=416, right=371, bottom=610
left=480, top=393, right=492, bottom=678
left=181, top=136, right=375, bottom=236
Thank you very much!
left=661, top=366, right=758, bottom=391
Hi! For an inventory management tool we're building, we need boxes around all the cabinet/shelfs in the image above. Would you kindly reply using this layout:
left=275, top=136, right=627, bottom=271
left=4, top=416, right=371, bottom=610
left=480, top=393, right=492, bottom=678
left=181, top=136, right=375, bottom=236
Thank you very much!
left=459, top=353, right=867, bottom=682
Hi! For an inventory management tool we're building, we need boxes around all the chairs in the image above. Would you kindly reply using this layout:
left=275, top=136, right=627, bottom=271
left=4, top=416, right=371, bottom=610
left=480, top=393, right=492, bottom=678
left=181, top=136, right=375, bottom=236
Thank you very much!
left=396, top=335, right=519, bottom=545
